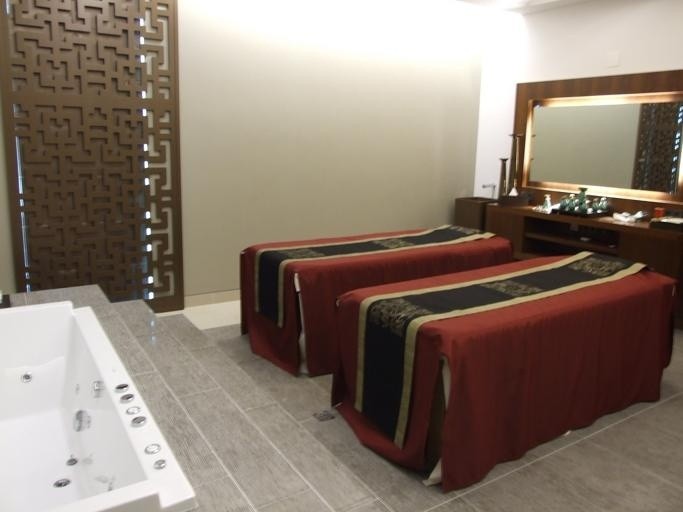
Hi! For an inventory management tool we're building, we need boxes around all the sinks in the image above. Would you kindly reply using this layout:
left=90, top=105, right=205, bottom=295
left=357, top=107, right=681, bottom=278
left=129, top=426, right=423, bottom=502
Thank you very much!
left=0, top=300, right=201, bottom=511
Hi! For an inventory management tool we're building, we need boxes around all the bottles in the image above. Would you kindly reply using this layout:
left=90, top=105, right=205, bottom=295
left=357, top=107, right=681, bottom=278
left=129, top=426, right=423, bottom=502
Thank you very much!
left=560, top=188, right=607, bottom=212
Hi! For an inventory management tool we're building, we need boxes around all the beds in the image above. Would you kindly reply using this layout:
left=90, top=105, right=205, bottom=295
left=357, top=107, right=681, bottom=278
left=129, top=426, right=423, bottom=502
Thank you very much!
left=240, top=224, right=513, bottom=378
left=330, top=251, right=678, bottom=491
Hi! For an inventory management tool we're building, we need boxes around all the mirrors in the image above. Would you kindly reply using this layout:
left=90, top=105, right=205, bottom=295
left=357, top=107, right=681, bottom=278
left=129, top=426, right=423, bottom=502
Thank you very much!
left=511, top=68, right=683, bottom=218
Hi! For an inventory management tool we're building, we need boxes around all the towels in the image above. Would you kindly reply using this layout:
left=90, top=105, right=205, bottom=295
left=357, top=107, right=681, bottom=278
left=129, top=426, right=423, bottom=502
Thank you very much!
left=625, top=210, right=649, bottom=223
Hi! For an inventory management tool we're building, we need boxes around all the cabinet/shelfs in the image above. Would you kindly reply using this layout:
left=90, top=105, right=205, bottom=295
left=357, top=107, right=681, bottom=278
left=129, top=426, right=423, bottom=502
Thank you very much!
left=485, top=202, right=683, bottom=329
